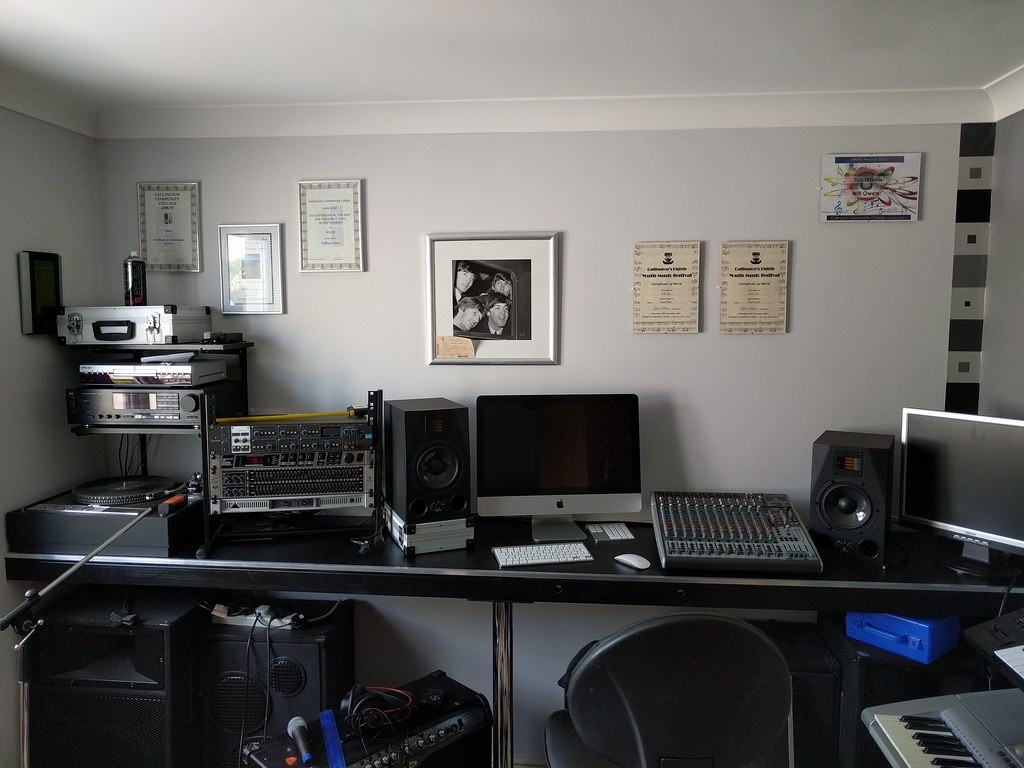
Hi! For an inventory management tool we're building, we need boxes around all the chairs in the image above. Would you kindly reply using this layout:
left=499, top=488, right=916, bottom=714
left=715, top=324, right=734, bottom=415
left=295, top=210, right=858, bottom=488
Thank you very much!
left=542, top=610, right=797, bottom=768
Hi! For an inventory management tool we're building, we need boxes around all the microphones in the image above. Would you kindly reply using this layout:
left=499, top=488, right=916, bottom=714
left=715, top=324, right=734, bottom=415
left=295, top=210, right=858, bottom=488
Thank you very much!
left=287, top=716, right=313, bottom=767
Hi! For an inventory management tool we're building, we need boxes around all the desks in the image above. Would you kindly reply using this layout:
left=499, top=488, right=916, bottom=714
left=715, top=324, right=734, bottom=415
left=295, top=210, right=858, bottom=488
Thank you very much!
left=4, top=544, right=1024, bottom=768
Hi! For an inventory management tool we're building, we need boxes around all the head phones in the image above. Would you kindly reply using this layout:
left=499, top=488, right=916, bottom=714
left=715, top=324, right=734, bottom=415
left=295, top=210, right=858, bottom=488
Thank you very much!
left=340, top=683, right=412, bottom=740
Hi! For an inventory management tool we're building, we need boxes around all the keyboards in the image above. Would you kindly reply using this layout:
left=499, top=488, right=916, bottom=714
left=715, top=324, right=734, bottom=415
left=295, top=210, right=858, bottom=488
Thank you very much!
left=586, top=523, right=636, bottom=542
left=492, top=542, right=593, bottom=568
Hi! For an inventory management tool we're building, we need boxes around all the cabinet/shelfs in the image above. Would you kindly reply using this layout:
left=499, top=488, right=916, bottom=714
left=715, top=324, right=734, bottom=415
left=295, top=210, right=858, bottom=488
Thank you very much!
left=58, top=341, right=255, bottom=558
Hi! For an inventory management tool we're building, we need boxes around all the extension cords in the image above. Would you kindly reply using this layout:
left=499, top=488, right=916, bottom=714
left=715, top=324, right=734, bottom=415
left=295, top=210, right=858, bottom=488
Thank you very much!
left=210, top=605, right=300, bottom=629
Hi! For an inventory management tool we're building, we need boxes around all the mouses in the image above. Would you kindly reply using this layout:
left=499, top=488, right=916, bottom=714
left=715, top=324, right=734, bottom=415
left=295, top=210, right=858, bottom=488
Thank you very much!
left=614, top=554, right=651, bottom=569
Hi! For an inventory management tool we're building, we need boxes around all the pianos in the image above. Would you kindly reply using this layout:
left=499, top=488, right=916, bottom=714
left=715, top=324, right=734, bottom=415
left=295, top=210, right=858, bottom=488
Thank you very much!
left=858, top=688, right=1024, bottom=768
left=964, top=606, right=1024, bottom=688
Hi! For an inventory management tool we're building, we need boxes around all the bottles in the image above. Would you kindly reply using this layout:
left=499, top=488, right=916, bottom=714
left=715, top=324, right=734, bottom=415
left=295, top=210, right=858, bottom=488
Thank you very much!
left=123, top=251, right=147, bottom=306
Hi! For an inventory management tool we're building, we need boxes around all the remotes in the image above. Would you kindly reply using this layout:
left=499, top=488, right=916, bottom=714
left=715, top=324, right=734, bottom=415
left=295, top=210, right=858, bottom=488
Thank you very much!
left=140, top=351, right=198, bottom=363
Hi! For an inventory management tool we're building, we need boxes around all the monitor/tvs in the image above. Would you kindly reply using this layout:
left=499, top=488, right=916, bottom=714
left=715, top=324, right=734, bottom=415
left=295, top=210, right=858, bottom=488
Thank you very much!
left=476, top=394, right=640, bottom=544
left=898, top=409, right=1024, bottom=579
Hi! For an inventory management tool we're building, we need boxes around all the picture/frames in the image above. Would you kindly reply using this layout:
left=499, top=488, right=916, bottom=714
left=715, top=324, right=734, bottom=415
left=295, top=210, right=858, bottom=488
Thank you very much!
left=426, top=230, right=560, bottom=365
left=297, top=180, right=364, bottom=272
left=217, top=224, right=285, bottom=315
left=137, top=182, right=202, bottom=272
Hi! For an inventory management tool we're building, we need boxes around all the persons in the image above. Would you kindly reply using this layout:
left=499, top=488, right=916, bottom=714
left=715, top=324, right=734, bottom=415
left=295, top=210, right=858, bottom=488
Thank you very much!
left=453, top=297, right=486, bottom=331
left=470, top=292, right=511, bottom=335
left=479, top=273, right=512, bottom=306
left=453, top=261, right=477, bottom=315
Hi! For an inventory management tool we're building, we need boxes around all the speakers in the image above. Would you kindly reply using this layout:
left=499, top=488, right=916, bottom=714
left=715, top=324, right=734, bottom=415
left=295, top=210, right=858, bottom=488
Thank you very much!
left=382, top=397, right=472, bottom=523
left=807, top=430, right=895, bottom=566
left=24, top=598, right=493, bottom=768
